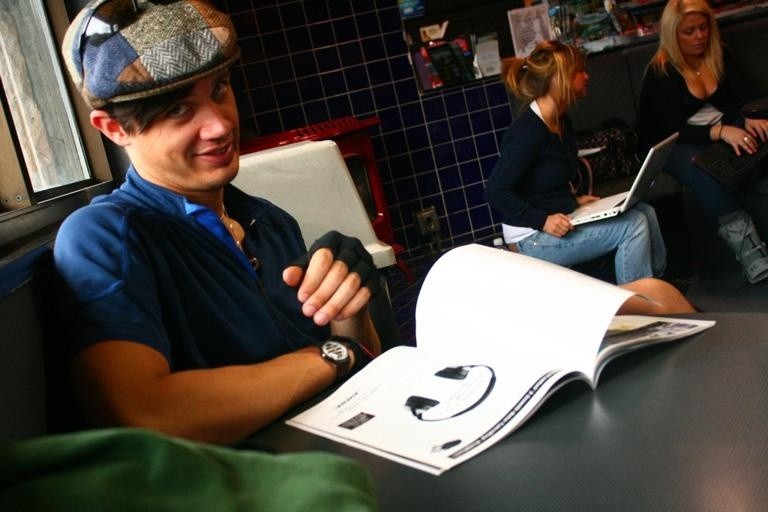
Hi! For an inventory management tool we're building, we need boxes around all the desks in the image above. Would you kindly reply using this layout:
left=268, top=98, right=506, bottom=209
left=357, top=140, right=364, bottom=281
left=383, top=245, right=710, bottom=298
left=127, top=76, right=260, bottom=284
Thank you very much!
left=226, top=313, right=768, bottom=512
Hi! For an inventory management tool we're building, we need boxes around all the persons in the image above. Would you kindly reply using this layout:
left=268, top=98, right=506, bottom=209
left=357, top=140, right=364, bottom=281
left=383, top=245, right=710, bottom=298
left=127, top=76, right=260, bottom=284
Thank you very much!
left=483, top=40, right=671, bottom=283
left=51, top=0, right=387, bottom=449
left=638, top=0, right=768, bottom=287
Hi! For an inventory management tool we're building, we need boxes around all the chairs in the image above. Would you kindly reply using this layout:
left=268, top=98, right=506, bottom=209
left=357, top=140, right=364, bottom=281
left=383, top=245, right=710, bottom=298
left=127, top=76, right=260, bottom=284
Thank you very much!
left=230, top=137, right=397, bottom=275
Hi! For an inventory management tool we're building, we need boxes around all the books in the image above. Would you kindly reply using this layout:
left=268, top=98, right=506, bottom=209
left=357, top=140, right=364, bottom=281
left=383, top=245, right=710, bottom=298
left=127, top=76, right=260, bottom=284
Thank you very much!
left=281, top=241, right=717, bottom=478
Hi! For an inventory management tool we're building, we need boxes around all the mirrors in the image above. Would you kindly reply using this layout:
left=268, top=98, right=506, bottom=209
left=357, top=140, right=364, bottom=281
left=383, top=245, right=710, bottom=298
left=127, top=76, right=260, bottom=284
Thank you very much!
left=398, top=0, right=750, bottom=96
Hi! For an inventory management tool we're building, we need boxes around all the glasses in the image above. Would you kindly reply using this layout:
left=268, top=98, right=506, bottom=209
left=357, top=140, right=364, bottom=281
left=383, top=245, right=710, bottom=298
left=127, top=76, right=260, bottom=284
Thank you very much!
left=79, top=0, right=181, bottom=79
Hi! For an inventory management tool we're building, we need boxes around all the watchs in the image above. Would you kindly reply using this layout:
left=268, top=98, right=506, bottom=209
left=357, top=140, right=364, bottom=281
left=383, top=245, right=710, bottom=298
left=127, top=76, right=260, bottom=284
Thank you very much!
left=316, top=337, right=352, bottom=383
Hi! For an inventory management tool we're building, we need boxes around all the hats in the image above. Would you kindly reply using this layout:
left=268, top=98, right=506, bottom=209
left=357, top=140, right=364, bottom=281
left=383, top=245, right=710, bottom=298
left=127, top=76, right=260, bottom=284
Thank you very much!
left=61, top=0, right=242, bottom=110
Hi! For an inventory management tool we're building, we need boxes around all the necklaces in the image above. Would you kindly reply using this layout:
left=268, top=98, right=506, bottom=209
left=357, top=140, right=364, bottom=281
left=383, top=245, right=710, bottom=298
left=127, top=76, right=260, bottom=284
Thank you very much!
left=220, top=208, right=260, bottom=271
left=695, top=70, right=703, bottom=77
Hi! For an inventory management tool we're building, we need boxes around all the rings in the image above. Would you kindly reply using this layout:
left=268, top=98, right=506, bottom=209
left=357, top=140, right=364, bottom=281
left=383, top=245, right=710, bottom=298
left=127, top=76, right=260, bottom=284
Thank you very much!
left=743, top=136, right=748, bottom=143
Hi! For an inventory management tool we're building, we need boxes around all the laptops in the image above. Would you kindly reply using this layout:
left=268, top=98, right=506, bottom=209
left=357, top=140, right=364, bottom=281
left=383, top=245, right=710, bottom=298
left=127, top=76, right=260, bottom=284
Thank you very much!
left=564, top=131, right=681, bottom=226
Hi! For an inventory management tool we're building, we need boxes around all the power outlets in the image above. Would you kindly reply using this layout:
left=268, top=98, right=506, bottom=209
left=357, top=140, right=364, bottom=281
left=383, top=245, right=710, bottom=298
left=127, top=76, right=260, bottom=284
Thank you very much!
left=416, top=209, right=440, bottom=234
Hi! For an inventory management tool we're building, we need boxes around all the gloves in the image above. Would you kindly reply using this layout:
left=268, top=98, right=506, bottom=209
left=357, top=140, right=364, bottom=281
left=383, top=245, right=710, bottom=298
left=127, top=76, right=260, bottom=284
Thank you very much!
left=289, top=230, right=381, bottom=303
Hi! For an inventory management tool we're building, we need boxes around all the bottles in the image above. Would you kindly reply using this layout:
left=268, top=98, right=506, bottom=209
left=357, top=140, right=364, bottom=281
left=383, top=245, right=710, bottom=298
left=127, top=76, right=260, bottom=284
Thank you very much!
left=493, top=237, right=509, bottom=251
